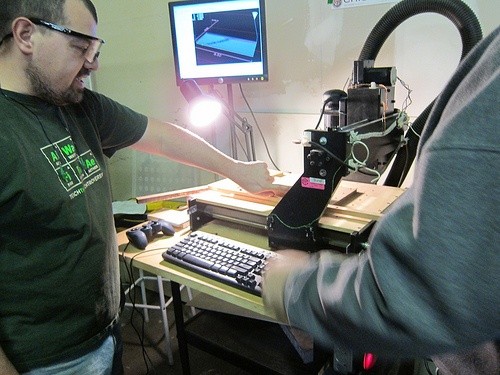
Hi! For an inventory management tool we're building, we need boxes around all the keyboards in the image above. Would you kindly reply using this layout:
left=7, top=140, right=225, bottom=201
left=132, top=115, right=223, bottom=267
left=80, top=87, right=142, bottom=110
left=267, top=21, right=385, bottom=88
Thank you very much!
left=162, top=230, right=277, bottom=296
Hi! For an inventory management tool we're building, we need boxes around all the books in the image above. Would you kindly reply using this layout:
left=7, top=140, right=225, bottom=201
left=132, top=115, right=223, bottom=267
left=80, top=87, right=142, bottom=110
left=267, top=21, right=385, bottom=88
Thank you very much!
left=146, top=209, right=192, bottom=229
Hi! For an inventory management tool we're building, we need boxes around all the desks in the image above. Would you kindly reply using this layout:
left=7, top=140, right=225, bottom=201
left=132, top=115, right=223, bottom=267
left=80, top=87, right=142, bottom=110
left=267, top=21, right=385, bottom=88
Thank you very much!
left=117, top=218, right=336, bottom=375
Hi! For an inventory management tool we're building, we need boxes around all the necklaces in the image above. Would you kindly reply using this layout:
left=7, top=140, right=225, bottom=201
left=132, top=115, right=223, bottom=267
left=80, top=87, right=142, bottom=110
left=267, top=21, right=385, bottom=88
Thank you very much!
left=1, top=88, right=91, bottom=176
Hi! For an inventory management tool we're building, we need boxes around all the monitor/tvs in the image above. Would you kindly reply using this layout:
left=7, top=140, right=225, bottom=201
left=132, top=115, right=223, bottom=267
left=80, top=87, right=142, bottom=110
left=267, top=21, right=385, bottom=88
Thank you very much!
left=168, top=0, right=268, bottom=86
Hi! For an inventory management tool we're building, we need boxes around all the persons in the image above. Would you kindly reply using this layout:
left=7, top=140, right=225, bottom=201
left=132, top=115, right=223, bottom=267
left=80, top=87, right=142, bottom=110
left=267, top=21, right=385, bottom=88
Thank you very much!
left=260, top=24, right=500, bottom=375
left=1, top=0, right=298, bottom=375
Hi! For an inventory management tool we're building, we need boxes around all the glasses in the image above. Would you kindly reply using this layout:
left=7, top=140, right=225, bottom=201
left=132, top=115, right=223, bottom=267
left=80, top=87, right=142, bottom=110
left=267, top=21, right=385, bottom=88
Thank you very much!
left=0, top=16, right=105, bottom=63
